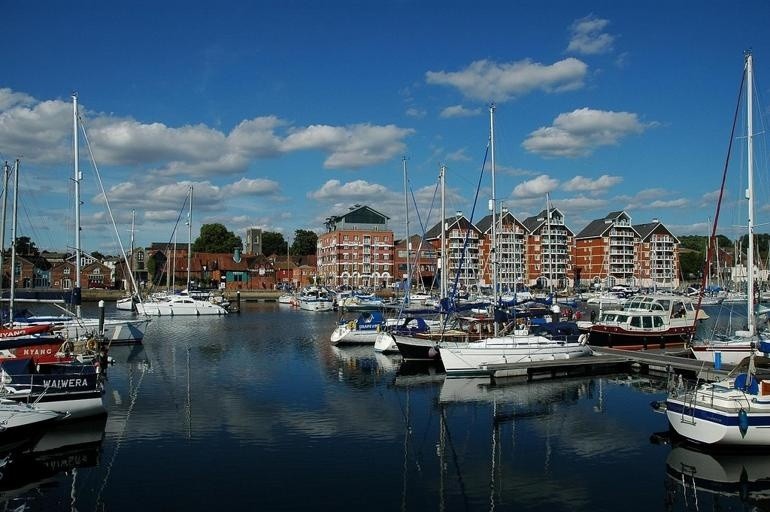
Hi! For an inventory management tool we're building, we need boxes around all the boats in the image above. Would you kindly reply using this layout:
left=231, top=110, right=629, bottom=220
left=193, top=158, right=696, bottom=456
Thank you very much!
left=3, top=443, right=98, bottom=501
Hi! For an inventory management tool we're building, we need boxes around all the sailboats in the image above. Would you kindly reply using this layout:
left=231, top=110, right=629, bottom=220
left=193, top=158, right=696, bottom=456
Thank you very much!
left=435, top=376, right=592, bottom=509
left=666, top=439, right=768, bottom=511
